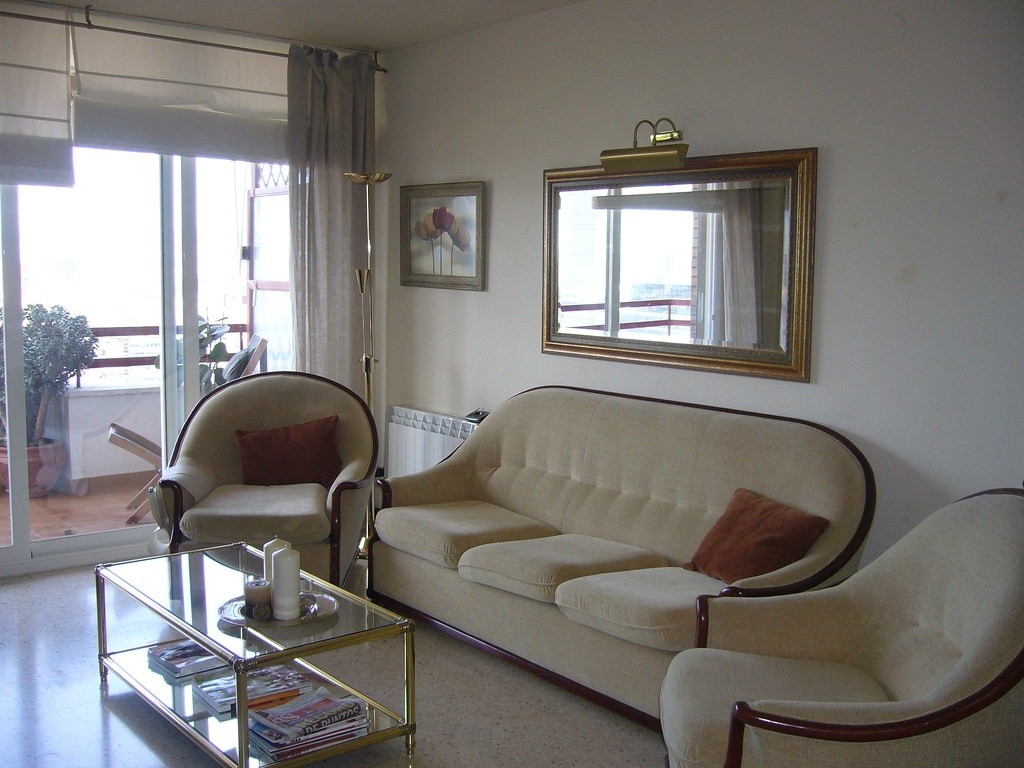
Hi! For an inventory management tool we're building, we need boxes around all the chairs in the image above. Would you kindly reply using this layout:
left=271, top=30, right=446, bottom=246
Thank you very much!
left=149, top=370, right=380, bottom=590
left=106, top=333, right=268, bottom=524
left=657, top=487, right=1024, bottom=768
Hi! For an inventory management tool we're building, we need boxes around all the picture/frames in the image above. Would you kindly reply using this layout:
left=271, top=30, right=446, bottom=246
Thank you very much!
left=398, top=180, right=488, bottom=292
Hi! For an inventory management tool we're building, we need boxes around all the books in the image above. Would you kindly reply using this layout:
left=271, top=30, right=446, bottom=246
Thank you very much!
left=192, top=663, right=312, bottom=713
left=148, top=640, right=227, bottom=677
left=250, top=714, right=370, bottom=761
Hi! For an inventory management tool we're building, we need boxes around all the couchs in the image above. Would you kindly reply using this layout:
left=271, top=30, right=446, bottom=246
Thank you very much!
left=365, top=384, right=876, bottom=737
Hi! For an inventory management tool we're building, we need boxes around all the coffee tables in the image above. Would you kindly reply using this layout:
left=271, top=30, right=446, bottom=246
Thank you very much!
left=94, top=538, right=417, bottom=768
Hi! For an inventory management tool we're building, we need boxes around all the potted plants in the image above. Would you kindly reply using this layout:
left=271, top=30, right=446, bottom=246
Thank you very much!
left=0, top=304, right=99, bottom=500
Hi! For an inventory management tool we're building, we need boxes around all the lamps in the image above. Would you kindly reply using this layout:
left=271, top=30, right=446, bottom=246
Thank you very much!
left=600, top=116, right=691, bottom=176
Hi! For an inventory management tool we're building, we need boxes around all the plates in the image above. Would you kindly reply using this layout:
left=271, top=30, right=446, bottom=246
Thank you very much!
left=220, top=593, right=338, bottom=627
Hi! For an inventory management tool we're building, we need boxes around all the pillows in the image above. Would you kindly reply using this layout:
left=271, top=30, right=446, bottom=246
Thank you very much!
left=683, top=489, right=831, bottom=586
left=233, top=414, right=344, bottom=489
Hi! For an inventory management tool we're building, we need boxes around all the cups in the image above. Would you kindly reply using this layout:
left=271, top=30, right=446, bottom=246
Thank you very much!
left=239, top=548, right=271, bottom=619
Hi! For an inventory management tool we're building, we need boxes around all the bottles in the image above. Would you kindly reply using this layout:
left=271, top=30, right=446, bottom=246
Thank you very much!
left=264, top=534, right=291, bottom=584
left=269, top=542, right=301, bottom=620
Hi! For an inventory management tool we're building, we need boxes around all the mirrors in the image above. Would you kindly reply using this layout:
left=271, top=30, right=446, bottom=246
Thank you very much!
left=540, top=144, right=819, bottom=387
